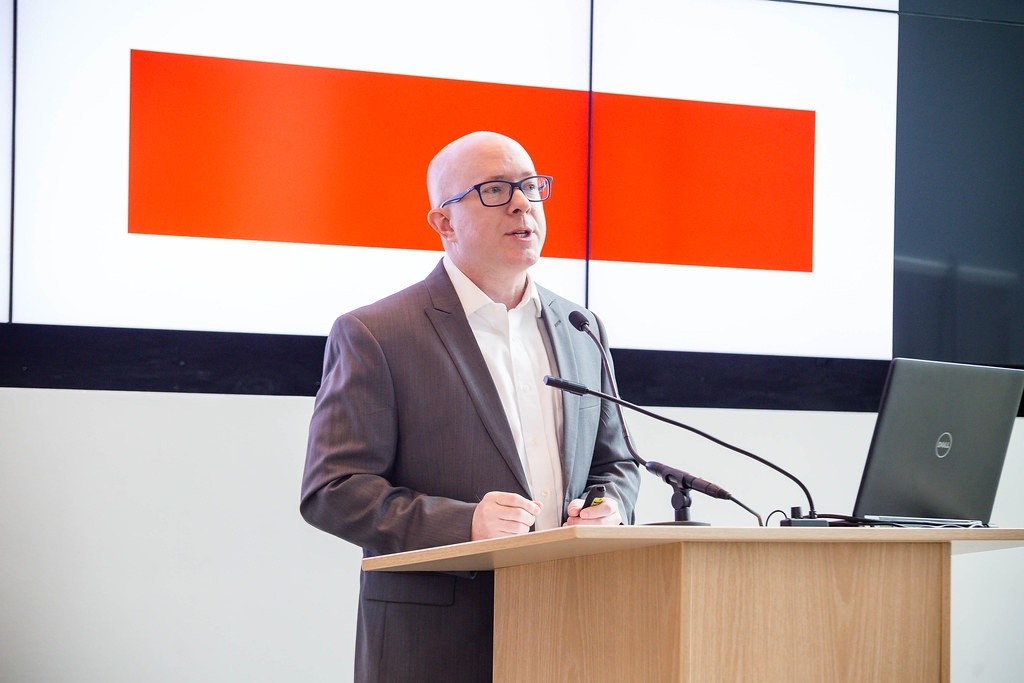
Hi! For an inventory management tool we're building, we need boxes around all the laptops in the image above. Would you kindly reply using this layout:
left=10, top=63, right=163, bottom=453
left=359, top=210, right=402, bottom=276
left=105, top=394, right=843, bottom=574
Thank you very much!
left=781, top=357, right=1024, bottom=527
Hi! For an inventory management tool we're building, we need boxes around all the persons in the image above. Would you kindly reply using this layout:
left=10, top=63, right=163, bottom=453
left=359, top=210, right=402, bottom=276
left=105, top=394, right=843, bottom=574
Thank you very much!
left=299, top=130, right=641, bottom=683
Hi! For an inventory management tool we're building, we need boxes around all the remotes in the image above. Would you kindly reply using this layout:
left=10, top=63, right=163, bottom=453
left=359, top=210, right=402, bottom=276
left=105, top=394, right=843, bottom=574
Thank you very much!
left=579, top=485, right=607, bottom=518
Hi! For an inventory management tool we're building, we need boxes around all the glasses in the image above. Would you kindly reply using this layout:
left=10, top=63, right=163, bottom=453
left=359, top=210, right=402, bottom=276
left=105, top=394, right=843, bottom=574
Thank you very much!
left=440, top=175, right=553, bottom=208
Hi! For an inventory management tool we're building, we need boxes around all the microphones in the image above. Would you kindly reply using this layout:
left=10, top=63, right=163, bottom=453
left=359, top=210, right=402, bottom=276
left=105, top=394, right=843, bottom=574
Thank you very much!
left=568, top=310, right=732, bottom=502
left=543, top=375, right=829, bottom=527
